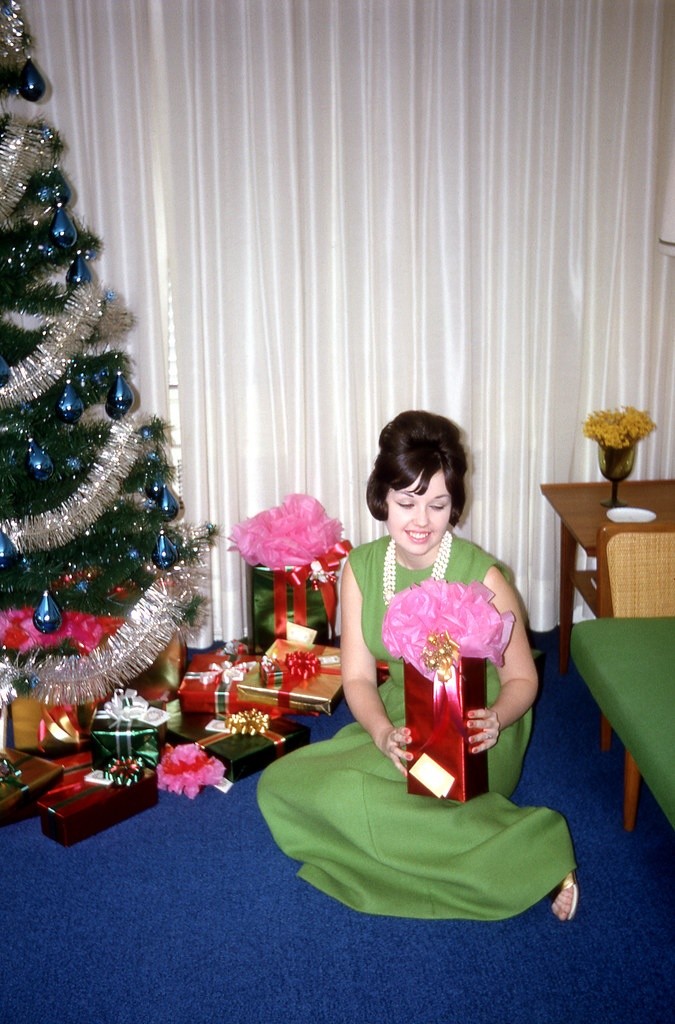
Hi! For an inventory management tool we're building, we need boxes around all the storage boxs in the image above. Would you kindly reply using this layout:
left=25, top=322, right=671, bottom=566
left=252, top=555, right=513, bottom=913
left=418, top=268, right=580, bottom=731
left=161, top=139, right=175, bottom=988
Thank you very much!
left=0, top=563, right=343, bottom=846
left=403, top=659, right=489, bottom=803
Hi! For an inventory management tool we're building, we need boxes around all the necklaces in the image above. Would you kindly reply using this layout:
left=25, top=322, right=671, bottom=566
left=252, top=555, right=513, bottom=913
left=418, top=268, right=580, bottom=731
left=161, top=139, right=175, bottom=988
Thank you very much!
left=382, top=532, right=454, bottom=615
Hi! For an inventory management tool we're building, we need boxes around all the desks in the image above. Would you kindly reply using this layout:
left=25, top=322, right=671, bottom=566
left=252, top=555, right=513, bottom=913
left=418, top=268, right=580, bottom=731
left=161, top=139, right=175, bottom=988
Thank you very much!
left=541, top=479, right=675, bottom=752
left=571, top=617, right=675, bottom=829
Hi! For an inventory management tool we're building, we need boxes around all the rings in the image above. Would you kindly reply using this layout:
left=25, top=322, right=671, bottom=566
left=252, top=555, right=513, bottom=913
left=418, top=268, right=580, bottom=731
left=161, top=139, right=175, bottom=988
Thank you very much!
left=483, top=733, right=492, bottom=740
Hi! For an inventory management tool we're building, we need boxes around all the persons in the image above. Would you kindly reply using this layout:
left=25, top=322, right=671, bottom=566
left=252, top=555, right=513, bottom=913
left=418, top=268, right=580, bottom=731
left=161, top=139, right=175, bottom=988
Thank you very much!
left=256, top=410, right=580, bottom=921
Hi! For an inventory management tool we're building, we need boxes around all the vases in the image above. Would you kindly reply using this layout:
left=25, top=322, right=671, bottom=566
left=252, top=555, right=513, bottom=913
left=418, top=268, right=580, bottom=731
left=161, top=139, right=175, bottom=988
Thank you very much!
left=599, top=444, right=635, bottom=508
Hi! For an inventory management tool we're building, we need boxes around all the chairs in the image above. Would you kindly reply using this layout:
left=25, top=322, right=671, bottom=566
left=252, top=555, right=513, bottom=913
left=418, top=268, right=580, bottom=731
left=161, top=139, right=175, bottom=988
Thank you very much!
left=592, top=522, right=675, bottom=832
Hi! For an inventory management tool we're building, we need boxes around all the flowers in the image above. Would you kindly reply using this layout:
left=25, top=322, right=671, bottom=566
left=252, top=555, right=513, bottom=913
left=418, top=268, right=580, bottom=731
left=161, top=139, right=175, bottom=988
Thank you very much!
left=383, top=577, right=516, bottom=683
left=157, top=744, right=227, bottom=799
left=582, top=406, right=657, bottom=473
left=229, top=493, right=345, bottom=569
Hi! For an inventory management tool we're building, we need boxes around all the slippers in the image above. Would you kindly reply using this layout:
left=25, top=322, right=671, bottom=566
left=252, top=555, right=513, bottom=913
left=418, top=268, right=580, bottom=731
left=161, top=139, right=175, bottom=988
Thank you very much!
left=547, top=871, right=579, bottom=921
